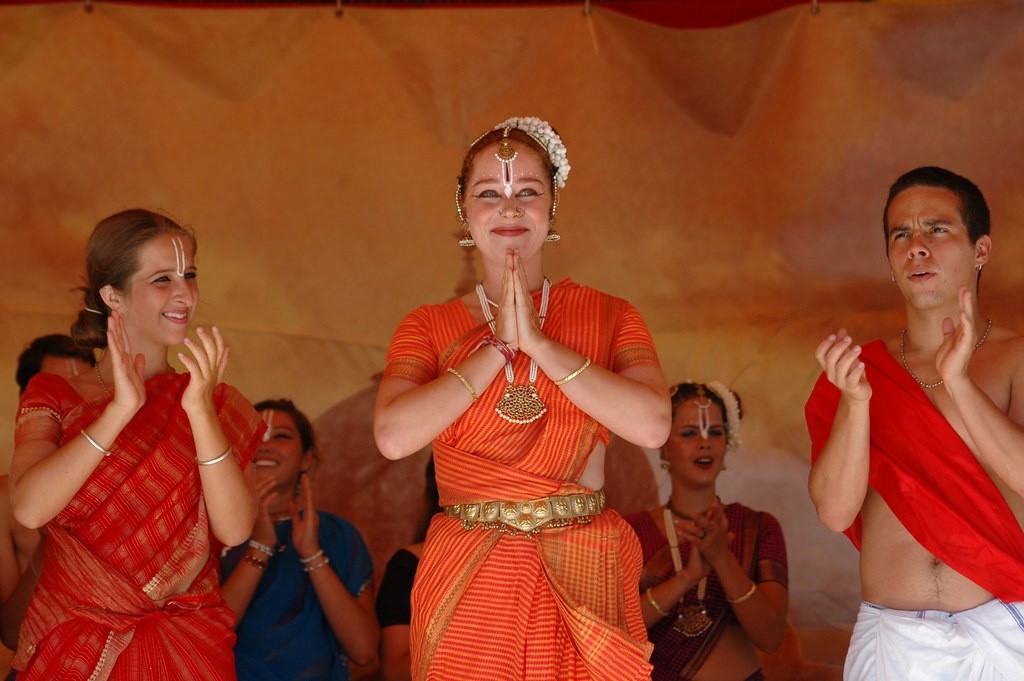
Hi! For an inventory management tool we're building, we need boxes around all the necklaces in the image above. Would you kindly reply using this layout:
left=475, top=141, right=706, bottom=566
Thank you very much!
left=95, top=363, right=108, bottom=392
left=663, top=494, right=728, bottom=637
left=474, top=275, right=553, bottom=424
left=267, top=509, right=287, bottom=514
left=900, top=315, right=992, bottom=389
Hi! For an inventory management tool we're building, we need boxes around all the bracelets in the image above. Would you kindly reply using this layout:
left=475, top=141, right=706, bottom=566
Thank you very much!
left=446, top=368, right=478, bottom=404
left=299, top=550, right=330, bottom=572
left=726, top=581, right=756, bottom=604
left=81, top=430, right=111, bottom=457
left=468, top=334, right=515, bottom=362
left=646, top=570, right=691, bottom=616
left=195, top=446, right=232, bottom=466
left=554, top=357, right=592, bottom=385
left=240, top=540, right=273, bottom=570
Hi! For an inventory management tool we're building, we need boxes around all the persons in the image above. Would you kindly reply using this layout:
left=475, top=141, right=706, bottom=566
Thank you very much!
left=9, top=208, right=268, bottom=681
left=373, top=114, right=672, bottom=681
left=0, top=334, right=103, bottom=681
left=805, top=164, right=1024, bottom=681
left=622, top=380, right=789, bottom=681
left=218, top=398, right=382, bottom=681
left=373, top=451, right=441, bottom=681
